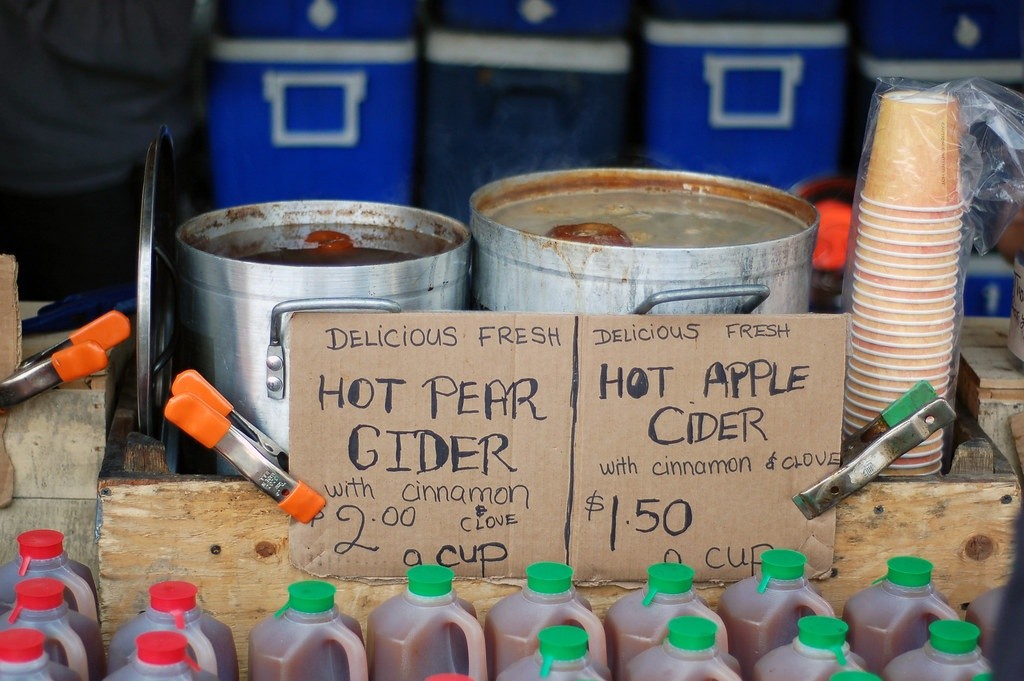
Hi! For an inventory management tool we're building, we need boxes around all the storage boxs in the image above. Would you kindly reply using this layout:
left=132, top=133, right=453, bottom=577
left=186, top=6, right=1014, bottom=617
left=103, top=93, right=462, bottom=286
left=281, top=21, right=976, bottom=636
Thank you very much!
left=0, top=0, right=1024, bottom=681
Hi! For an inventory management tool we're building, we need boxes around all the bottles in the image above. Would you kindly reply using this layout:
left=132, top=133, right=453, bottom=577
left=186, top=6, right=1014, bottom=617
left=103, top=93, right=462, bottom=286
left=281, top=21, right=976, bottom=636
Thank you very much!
left=1, top=532, right=1024, bottom=681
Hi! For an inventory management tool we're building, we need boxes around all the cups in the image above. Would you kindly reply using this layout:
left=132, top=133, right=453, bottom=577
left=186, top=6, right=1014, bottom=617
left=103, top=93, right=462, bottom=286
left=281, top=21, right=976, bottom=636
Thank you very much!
left=842, top=90, right=963, bottom=478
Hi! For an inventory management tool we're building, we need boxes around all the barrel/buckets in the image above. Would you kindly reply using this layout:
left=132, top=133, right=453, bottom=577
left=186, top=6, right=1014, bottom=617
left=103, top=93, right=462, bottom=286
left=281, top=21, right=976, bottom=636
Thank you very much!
left=173, top=200, right=472, bottom=476
left=469, top=168, right=820, bottom=314
